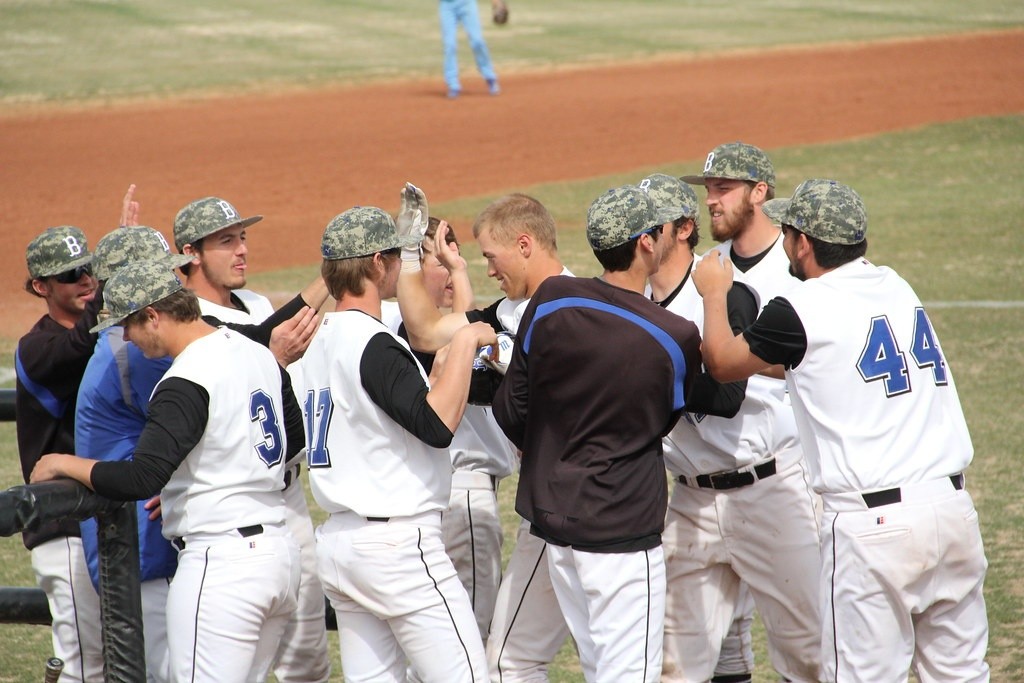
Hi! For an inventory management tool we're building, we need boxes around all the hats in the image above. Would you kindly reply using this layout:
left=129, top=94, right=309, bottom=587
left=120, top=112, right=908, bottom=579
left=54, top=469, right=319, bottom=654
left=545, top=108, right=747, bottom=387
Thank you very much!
left=321, top=205, right=426, bottom=261
left=175, top=196, right=264, bottom=251
left=88, top=257, right=182, bottom=332
left=93, top=224, right=196, bottom=281
left=586, top=184, right=684, bottom=251
left=636, top=173, right=700, bottom=220
left=26, top=226, right=94, bottom=279
left=678, top=141, right=776, bottom=187
left=761, top=179, right=867, bottom=245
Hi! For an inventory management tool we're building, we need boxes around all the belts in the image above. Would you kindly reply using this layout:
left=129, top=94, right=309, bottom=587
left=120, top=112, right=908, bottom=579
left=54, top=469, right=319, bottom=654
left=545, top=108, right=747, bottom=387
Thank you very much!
left=490, top=474, right=500, bottom=491
left=862, top=475, right=962, bottom=509
left=678, top=458, right=775, bottom=489
left=366, top=517, right=390, bottom=522
left=174, top=524, right=264, bottom=550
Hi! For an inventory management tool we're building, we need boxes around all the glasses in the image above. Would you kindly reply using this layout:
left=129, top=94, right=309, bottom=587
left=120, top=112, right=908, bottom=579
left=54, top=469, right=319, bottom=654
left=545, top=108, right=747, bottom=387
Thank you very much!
left=627, top=225, right=664, bottom=239
left=781, top=223, right=797, bottom=235
left=54, top=262, right=93, bottom=283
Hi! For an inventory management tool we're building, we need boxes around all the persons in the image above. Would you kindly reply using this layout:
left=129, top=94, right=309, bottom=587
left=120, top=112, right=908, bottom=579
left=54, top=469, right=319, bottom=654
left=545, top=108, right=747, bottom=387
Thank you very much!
left=14, top=142, right=991, bottom=683
left=439, top=0, right=509, bottom=99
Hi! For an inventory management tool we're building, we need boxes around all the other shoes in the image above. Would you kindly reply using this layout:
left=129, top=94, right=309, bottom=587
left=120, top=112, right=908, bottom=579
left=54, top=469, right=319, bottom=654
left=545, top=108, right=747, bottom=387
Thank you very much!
left=448, top=90, right=457, bottom=99
left=487, top=80, right=499, bottom=95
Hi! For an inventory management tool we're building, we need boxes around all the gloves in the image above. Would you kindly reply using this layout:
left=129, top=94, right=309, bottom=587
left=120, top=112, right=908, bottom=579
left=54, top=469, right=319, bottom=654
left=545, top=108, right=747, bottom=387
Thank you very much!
left=396, top=182, right=428, bottom=272
left=478, top=330, right=515, bottom=377
